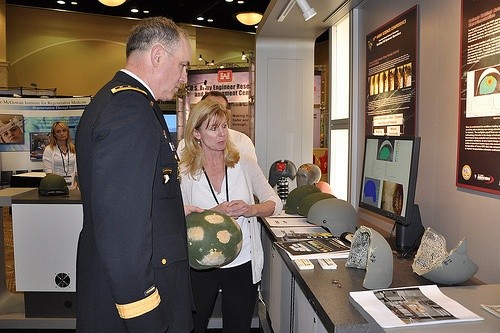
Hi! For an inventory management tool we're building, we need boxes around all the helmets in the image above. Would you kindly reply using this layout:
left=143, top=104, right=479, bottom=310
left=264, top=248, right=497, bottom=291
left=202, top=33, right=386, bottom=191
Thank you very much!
left=345, top=226, right=393, bottom=289
left=307, top=198, right=359, bottom=237
left=298, top=192, right=337, bottom=215
left=185, top=209, right=243, bottom=270
left=296, top=164, right=321, bottom=186
left=286, top=185, right=321, bottom=213
left=269, top=160, right=296, bottom=188
left=412, top=227, right=478, bottom=284
left=39, top=173, right=69, bottom=195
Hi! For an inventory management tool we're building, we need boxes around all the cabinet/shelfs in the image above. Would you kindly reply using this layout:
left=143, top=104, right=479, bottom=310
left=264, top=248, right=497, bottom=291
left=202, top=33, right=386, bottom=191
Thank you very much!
left=257, top=210, right=488, bottom=333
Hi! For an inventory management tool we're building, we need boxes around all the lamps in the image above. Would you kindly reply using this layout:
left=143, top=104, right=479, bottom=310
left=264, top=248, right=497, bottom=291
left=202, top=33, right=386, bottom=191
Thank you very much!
left=98, top=0, right=126, bottom=7
left=241, top=52, right=246, bottom=60
left=295, top=0, right=317, bottom=21
left=234, top=11, right=263, bottom=25
left=199, top=54, right=215, bottom=66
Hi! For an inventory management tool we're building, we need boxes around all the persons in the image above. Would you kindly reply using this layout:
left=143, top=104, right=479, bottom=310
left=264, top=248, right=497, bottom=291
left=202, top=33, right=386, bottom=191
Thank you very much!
left=0, top=120, right=24, bottom=143
left=176, top=100, right=283, bottom=332
left=43, top=122, right=78, bottom=187
left=177, top=90, right=257, bottom=162
left=75, top=17, right=194, bottom=333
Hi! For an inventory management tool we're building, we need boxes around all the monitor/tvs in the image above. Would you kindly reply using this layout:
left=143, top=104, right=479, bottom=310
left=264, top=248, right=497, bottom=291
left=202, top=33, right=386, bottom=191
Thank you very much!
left=359, top=135, right=425, bottom=259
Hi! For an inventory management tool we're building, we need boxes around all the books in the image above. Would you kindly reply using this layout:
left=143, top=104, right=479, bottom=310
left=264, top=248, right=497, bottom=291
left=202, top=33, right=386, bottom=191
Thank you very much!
left=273, top=237, right=350, bottom=261
